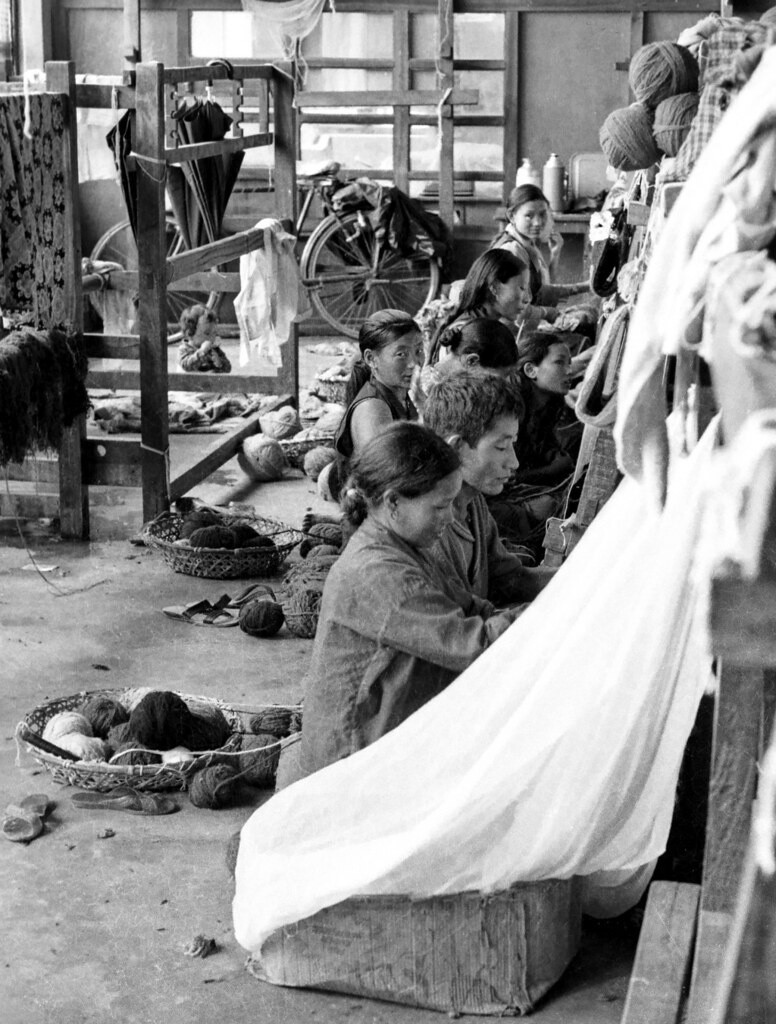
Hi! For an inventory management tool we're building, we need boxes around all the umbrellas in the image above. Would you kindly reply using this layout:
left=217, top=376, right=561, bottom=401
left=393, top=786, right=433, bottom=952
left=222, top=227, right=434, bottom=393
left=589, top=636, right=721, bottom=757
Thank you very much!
left=108, top=58, right=244, bottom=250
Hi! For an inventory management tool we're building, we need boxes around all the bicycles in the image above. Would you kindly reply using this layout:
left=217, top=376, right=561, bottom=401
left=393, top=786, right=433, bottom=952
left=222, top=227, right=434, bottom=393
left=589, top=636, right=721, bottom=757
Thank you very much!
left=90, top=161, right=438, bottom=343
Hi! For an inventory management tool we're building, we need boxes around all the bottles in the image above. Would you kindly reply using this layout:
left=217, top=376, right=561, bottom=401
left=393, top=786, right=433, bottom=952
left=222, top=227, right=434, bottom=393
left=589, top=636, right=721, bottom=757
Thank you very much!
left=515, top=157, right=541, bottom=189
left=543, top=153, right=565, bottom=214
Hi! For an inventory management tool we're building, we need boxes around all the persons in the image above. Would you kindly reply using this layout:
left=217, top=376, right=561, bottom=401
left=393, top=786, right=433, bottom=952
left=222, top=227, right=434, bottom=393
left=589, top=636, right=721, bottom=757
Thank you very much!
left=334, top=184, right=591, bottom=603
left=284, top=420, right=530, bottom=778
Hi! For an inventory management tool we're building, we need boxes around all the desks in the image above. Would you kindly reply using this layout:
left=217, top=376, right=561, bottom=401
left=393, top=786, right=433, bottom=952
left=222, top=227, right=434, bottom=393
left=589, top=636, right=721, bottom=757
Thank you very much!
left=493, top=211, right=592, bottom=235
